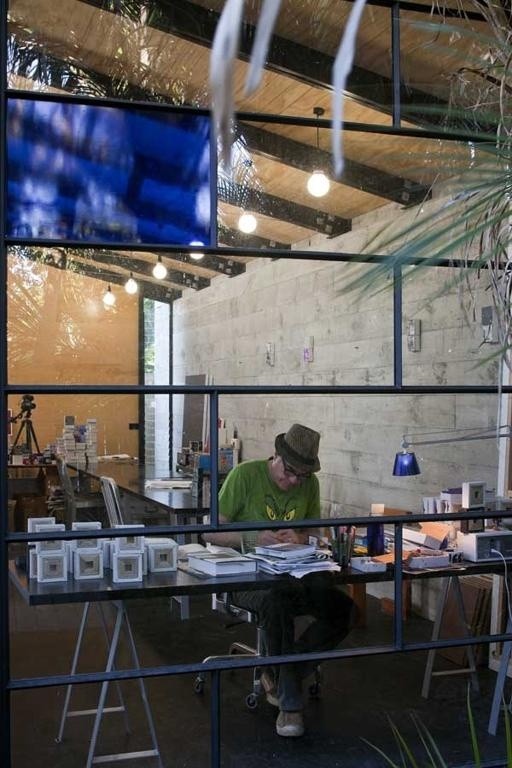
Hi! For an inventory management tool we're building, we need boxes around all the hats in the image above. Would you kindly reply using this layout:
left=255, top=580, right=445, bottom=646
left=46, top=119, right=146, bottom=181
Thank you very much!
left=274, top=422, right=321, bottom=472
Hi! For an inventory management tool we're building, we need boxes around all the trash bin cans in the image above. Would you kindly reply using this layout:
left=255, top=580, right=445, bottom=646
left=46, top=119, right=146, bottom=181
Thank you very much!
left=14, top=493, right=48, bottom=533
left=5, top=499, right=15, bottom=533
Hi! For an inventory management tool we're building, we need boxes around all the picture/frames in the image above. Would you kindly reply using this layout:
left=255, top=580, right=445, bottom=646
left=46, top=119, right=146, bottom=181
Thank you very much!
left=7, top=406, right=13, bottom=437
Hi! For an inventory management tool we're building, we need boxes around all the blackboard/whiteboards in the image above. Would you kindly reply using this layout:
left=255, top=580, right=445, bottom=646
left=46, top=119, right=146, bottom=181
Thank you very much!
left=181, top=374, right=207, bottom=449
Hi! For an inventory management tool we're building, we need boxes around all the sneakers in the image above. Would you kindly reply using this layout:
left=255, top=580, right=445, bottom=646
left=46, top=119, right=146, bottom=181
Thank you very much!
left=261, top=673, right=283, bottom=705
left=275, top=708, right=305, bottom=737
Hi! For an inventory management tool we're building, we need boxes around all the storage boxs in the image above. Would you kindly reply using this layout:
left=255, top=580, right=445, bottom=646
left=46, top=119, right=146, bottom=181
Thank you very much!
left=5, top=464, right=61, bottom=531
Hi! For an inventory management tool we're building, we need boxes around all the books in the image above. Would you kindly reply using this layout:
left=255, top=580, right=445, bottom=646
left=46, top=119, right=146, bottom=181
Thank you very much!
left=188, top=552, right=258, bottom=578
left=248, top=538, right=335, bottom=577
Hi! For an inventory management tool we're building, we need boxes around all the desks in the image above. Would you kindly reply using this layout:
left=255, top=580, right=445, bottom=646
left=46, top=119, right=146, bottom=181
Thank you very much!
left=5, top=552, right=511, bottom=768
left=63, top=459, right=221, bottom=619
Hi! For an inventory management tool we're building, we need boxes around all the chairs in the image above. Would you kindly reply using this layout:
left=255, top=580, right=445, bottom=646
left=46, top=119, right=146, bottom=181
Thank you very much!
left=190, top=467, right=327, bottom=709
left=56, top=458, right=106, bottom=526
left=100, top=475, right=126, bottom=528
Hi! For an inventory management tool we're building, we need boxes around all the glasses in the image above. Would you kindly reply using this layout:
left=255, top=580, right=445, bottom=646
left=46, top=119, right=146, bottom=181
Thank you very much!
left=280, top=455, right=311, bottom=482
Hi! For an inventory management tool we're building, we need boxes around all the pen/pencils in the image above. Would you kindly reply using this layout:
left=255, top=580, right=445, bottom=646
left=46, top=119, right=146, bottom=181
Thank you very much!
left=330, top=526, right=356, bottom=566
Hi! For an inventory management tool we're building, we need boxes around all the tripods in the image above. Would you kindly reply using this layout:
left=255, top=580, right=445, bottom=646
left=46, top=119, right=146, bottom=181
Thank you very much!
left=9, top=412, right=40, bottom=455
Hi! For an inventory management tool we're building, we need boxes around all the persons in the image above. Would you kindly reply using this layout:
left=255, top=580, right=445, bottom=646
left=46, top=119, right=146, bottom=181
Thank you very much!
left=201, top=424, right=361, bottom=737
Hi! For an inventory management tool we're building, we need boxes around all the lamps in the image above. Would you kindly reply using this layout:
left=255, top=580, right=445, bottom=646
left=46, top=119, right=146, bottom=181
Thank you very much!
left=391, top=423, right=511, bottom=477
